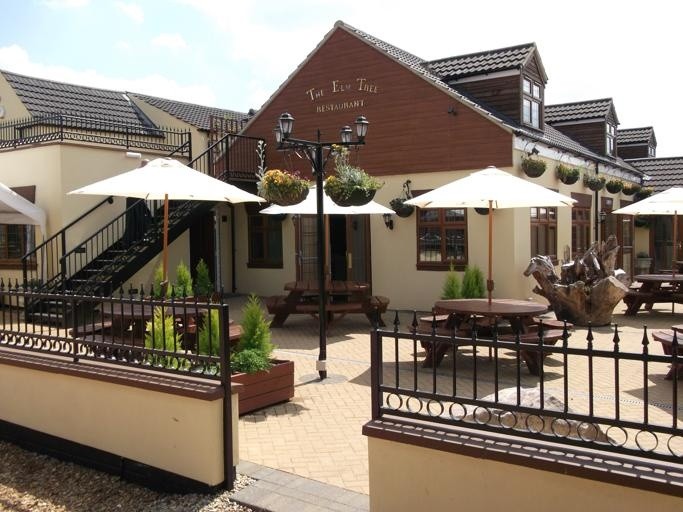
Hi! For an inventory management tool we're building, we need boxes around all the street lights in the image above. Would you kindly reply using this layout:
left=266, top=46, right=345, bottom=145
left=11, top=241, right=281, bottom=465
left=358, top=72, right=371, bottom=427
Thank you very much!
left=272, top=111, right=370, bottom=378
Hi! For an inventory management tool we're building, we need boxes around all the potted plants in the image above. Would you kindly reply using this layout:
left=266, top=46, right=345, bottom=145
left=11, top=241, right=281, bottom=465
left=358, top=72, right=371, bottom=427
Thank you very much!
left=144, top=297, right=294, bottom=415
left=583, top=174, right=654, bottom=197
left=388, top=196, right=414, bottom=218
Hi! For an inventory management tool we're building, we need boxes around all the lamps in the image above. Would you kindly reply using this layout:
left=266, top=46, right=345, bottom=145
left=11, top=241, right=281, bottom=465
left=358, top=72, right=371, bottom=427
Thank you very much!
left=382, top=213, right=393, bottom=229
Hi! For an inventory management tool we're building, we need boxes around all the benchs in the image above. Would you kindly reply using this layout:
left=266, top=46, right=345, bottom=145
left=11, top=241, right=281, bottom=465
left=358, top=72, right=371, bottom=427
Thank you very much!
left=265, top=294, right=390, bottom=331
left=416, top=315, right=574, bottom=378
left=651, top=327, right=683, bottom=381
left=69, top=318, right=245, bottom=356
left=621, top=286, right=683, bottom=317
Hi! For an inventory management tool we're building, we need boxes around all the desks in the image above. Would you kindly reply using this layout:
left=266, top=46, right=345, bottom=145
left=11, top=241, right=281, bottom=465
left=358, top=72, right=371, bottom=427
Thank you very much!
left=423, top=299, right=552, bottom=382
left=624, top=274, right=683, bottom=317
left=92, top=300, right=222, bottom=337
left=269, top=279, right=383, bottom=332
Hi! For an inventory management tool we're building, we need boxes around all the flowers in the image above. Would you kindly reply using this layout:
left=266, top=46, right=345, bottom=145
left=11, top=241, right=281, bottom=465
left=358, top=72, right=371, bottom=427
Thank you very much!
left=251, top=137, right=308, bottom=204
left=523, top=157, right=548, bottom=177
left=557, top=163, right=580, bottom=184
left=323, top=145, right=383, bottom=198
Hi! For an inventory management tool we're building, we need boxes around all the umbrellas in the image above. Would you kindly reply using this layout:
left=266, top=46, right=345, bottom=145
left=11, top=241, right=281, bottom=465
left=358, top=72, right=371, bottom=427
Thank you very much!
left=67, top=156, right=267, bottom=280
left=258, top=180, right=397, bottom=324
left=402, top=165, right=579, bottom=358
left=610, top=186, right=683, bottom=314
